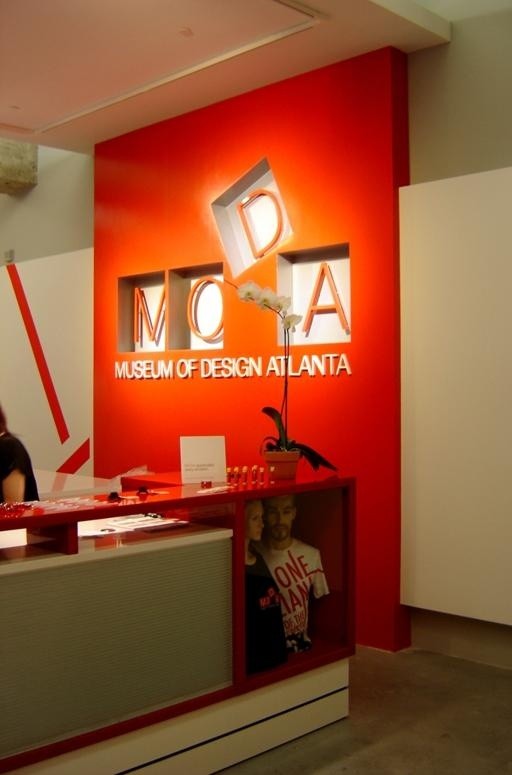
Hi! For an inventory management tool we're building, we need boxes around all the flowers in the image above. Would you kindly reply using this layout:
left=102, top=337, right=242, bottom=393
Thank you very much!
left=222, top=278, right=340, bottom=471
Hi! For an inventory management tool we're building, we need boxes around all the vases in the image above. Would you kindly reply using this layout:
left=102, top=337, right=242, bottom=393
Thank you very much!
left=263, top=452, right=301, bottom=481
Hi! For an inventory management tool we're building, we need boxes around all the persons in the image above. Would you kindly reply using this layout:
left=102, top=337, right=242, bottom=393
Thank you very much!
left=253, top=493, right=330, bottom=654
left=0, top=404, right=39, bottom=505
left=245, top=501, right=289, bottom=673
